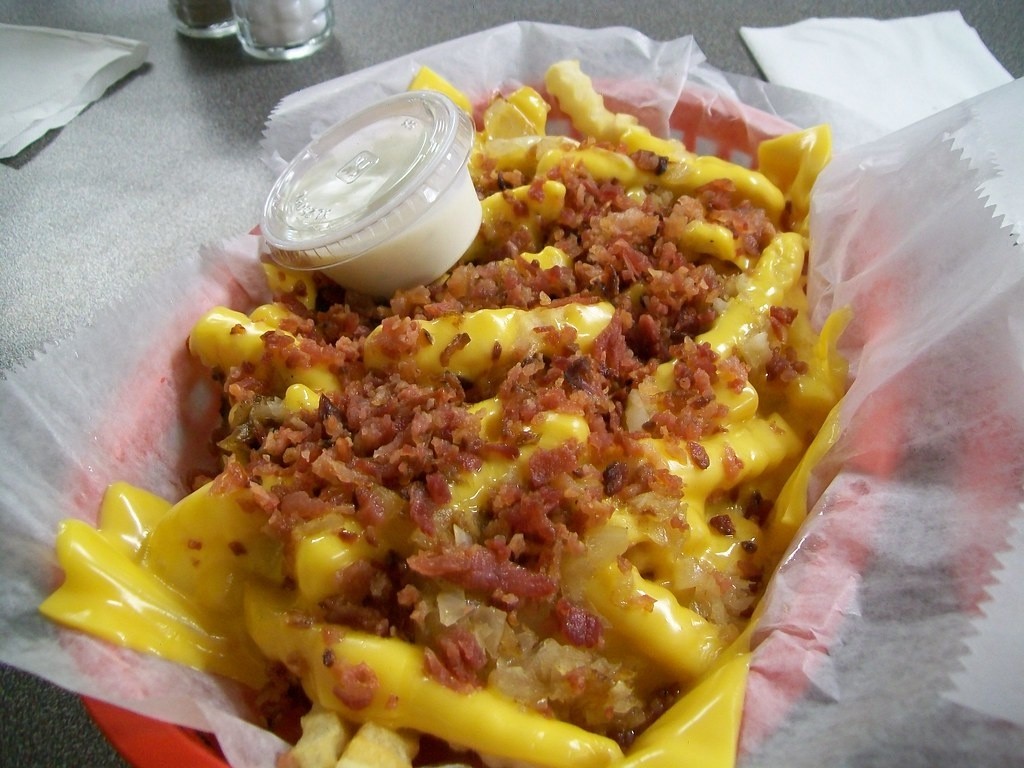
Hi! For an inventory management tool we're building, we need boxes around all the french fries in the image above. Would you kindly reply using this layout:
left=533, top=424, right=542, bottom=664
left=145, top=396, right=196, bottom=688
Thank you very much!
left=138, top=61, right=830, bottom=768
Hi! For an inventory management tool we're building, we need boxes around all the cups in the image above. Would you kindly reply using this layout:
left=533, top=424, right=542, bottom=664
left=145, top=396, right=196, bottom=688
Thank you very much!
left=231, top=0, right=335, bottom=61
left=167, top=0, right=237, bottom=39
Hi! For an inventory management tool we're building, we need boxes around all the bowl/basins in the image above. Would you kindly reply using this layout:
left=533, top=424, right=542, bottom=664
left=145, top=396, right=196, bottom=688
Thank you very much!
left=259, top=90, right=483, bottom=305
